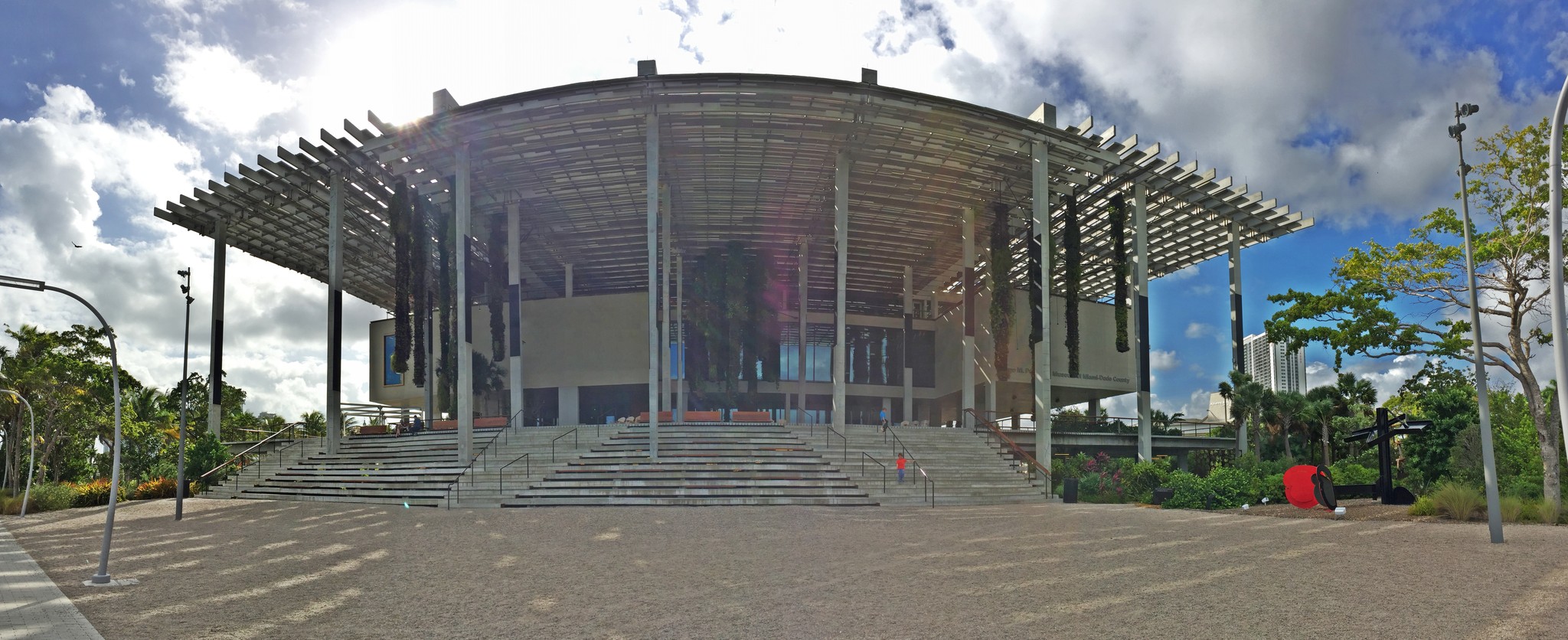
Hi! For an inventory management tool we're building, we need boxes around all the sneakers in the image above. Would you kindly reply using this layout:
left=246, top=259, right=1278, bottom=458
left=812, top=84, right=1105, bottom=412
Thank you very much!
left=413, top=432, right=418, bottom=437
left=881, top=427, right=884, bottom=431
left=398, top=435, right=400, bottom=437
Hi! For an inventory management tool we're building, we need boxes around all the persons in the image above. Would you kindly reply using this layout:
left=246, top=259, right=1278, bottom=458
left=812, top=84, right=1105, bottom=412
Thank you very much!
left=395, top=420, right=404, bottom=438
left=880, top=407, right=888, bottom=432
left=402, top=422, right=408, bottom=432
left=896, top=452, right=916, bottom=485
left=409, top=415, right=421, bottom=436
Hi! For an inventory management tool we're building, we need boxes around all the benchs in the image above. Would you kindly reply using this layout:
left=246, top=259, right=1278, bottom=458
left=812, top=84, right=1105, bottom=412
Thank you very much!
left=473, top=417, right=508, bottom=429
left=750, top=476, right=803, bottom=481
left=637, top=411, right=675, bottom=423
left=618, top=436, right=650, bottom=440
left=672, top=454, right=720, bottom=458
left=429, top=448, right=458, bottom=451
left=280, top=485, right=320, bottom=489
left=276, top=473, right=314, bottom=476
left=391, top=422, right=426, bottom=434
left=559, top=470, right=609, bottom=474
left=692, top=441, right=738, bottom=444
left=759, top=447, right=806, bottom=451
left=341, top=480, right=379, bottom=484
left=682, top=411, right=723, bottom=422
left=430, top=420, right=458, bottom=431
left=619, top=469, right=665, bottom=472
left=731, top=411, right=773, bottom=423
left=684, top=485, right=736, bottom=489
left=349, top=446, right=388, bottom=448
left=355, top=426, right=387, bottom=435
left=359, top=456, right=397, bottom=459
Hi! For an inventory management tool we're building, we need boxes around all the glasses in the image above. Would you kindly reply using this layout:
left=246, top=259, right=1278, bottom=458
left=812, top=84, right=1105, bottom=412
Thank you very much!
left=400, top=421, right=403, bottom=422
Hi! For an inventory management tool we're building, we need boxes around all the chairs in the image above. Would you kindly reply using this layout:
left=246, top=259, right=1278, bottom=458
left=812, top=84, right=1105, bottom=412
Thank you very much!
left=778, top=419, right=787, bottom=424
left=915, top=420, right=930, bottom=427
left=610, top=417, right=626, bottom=425
left=626, top=415, right=641, bottom=425
left=895, top=421, right=910, bottom=427
left=906, top=421, right=920, bottom=427
left=618, top=416, right=634, bottom=425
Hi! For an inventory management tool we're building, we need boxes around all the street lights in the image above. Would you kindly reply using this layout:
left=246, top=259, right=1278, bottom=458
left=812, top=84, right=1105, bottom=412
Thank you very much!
left=175, top=267, right=194, bottom=520
left=1447, top=102, right=1506, bottom=543
left=0, top=274, right=121, bottom=586
